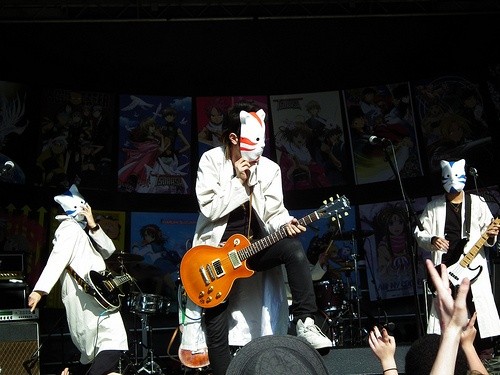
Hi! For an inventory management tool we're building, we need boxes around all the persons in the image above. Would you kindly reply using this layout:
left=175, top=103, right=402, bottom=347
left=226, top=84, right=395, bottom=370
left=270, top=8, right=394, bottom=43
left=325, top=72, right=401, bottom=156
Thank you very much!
left=192, top=101, right=333, bottom=375
left=31, top=184, right=130, bottom=375
left=369, top=258, right=489, bottom=375
left=414, top=159, right=500, bottom=360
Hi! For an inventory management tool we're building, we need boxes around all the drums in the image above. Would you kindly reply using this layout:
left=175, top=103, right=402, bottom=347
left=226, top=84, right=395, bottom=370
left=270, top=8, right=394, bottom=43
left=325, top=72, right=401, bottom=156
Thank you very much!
left=286, top=304, right=333, bottom=342
left=313, top=279, right=352, bottom=312
left=127, top=291, right=162, bottom=313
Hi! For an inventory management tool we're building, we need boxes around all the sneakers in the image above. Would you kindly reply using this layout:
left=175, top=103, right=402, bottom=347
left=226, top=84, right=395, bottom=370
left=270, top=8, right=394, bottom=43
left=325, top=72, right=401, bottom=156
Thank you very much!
left=296, top=317, right=333, bottom=349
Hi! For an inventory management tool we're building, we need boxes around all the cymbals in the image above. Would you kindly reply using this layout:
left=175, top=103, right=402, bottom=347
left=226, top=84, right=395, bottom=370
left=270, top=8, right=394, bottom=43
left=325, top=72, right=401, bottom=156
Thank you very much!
left=329, top=265, right=368, bottom=273
left=329, top=229, right=375, bottom=241
left=105, top=252, right=145, bottom=264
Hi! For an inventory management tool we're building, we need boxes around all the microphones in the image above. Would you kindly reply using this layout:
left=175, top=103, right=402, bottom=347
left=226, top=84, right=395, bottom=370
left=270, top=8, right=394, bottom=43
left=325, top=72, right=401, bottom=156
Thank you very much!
left=369, top=135, right=390, bottom=144
left=469, top=167, right=477, bottom=174
left=2, top=160, right=14, bottom=172
left=361, top=239, right=366, bottom=248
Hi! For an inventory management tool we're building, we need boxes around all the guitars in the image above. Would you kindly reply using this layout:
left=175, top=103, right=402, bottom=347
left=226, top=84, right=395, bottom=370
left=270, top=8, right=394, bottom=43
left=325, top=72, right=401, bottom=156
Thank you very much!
left=86, top=270, right=136, bottom=312
left=179, top=193, right=352, bottom=309
left=426, top=217, right=500, bottom=300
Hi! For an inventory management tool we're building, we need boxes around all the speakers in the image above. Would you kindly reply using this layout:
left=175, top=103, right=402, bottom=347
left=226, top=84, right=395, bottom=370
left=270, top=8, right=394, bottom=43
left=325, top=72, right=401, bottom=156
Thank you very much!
left=319, top=344, right=412, bottom=375
left=0, top=282, right=40, bottom=375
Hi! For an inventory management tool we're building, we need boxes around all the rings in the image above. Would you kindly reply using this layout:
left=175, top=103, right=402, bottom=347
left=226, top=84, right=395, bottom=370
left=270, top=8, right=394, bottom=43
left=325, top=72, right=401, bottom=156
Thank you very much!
left=434, top=291, right=437, bottom=296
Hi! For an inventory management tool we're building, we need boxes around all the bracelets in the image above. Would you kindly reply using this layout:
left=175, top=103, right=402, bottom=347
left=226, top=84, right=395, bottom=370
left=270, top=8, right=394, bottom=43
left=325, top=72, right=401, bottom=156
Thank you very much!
left=91, top=225, right=101, bottom=231
left=383, top=367, right=398, bottom=373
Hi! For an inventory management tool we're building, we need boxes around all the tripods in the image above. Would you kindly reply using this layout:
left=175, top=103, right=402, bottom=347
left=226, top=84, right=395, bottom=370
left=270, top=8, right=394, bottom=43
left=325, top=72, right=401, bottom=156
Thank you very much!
left=121, top=325, right=166, bottom=375
